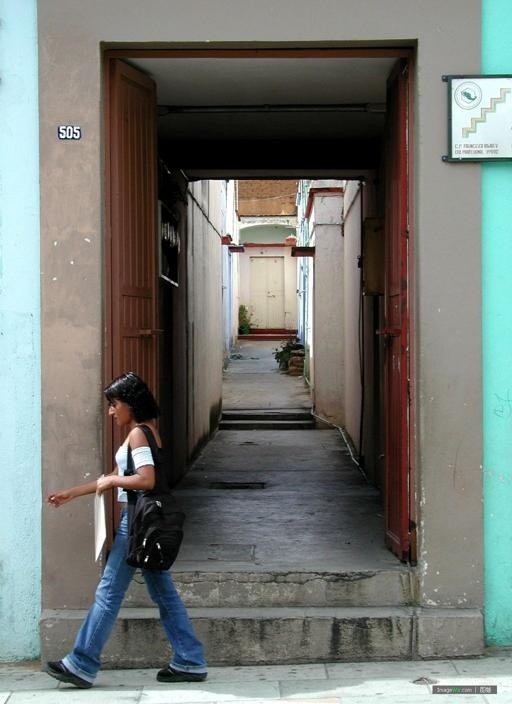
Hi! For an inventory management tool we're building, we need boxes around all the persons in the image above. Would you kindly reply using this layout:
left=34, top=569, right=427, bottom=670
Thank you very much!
left=45, top=371, right=207, bottom=690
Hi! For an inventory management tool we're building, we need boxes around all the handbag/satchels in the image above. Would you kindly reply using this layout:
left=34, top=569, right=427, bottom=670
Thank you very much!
left=123, top=423, right=185, bottom=572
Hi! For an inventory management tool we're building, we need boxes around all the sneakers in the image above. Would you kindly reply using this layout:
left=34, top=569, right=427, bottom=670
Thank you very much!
left=45, top=659, right=93, bottom=690
left=156, top=665, right=207, bottom=682
